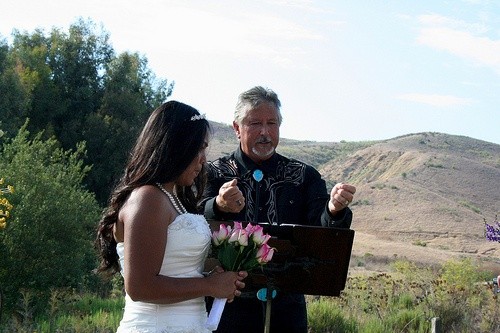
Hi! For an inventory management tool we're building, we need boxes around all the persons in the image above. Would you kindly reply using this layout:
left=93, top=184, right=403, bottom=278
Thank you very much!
left=92, top=100, right=248, bottom=333
left=192, top=87, right=357, bottom=333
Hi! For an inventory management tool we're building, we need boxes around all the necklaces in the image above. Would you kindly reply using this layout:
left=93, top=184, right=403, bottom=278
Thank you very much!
left=155, top=181, right=189, bottom=215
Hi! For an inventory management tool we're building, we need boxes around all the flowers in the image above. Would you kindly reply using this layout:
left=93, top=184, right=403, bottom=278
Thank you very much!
left=206, top=220, right=275, bottom=331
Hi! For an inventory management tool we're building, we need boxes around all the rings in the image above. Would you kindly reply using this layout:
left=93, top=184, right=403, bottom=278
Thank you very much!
left=236, top=200, right=242, bottom=206
left=342, top=200, right=349, bottom=206
